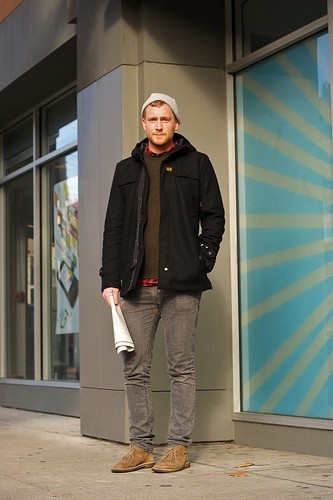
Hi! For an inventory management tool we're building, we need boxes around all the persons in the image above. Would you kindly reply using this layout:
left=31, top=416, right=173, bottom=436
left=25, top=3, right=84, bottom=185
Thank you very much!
left=99, top=93, right=225, bottom=474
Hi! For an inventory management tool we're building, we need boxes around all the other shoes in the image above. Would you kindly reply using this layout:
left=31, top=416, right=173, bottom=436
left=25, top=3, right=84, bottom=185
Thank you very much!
left=111, top=444, right=155, bottom=473
left=151, top=445, right=190, bottom=472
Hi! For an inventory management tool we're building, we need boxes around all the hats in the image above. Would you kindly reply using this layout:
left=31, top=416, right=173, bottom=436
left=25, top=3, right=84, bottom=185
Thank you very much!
left=141, top=93, right=181, bottom=125
